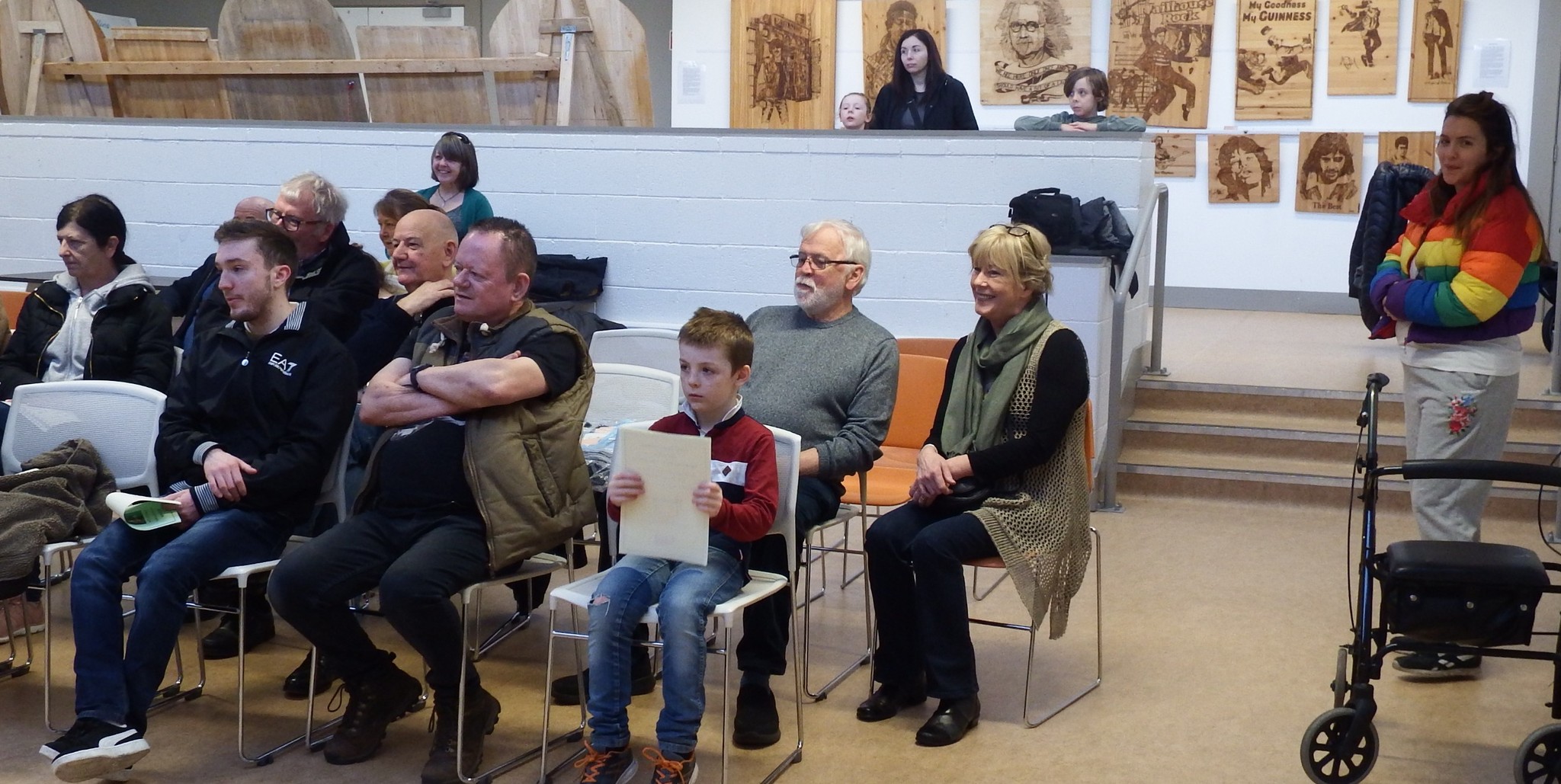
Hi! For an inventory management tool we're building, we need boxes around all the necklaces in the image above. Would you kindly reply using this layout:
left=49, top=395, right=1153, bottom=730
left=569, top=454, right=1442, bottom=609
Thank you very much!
left=438, top=187, right=462, bottom=207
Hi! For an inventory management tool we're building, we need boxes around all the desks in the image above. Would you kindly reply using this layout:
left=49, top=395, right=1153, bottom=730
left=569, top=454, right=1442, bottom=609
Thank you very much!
left=0, top=269, right=574, bottom=314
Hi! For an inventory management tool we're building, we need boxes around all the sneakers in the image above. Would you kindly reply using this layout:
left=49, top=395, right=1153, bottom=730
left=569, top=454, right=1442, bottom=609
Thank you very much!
left=574, top=741, right=639, bottom=784
left=52, top=720, right=151, bottom=782
left=0, top=595, right=45, bottom=643
left=39, top=717, right=133, bottom=771
left=183, top=589, right=233, bottom=623
left=323, top=650, right=426, bottom=764
left=551, top=654, right=657, bottom=700
left=643, top=747, right=700, bottom=784
left=420, top=669, right=501, bottom=784
left=1390, top=636, right=1482, bottom=676
left=732, top=680, right=781, bottom=751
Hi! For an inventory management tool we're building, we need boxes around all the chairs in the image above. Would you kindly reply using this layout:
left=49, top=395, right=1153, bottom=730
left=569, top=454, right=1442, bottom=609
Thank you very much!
left=304, top=326, right=690, bottom=784
left=0, top=380, right=185, bottom=737
left=122, top=410, right=354, bottom=768
left=869, top=399, right=1103, bottom=728
left=535, top=337, right=970, bottom=784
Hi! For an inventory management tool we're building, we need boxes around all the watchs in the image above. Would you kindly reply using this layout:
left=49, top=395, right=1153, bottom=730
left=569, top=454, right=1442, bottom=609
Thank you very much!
left=410, top=364, right=435, bottom=392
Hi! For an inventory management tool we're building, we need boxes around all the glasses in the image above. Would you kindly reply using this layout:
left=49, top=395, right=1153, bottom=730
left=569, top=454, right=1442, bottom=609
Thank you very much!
left=989, top=224, right=1039, bottom=259
left=265, top=208, right=324, bottom=231
left=442, top=131, right=469, bottom=143
left=789, top=254, right=858, bottom=270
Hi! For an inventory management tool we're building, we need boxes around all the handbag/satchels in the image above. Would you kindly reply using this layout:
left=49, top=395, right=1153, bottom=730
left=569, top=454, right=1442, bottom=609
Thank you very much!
left=1008, top=187, right=1072, bottom=245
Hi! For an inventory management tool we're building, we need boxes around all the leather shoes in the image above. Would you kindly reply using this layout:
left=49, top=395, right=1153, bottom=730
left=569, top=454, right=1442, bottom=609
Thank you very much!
left=856, top=684, right=927, bottom=721
left=915, top=692, right=981, bottom=745
left=202, top=600, right=276, bottom=659
left=283, top=647, right=339, bottom=699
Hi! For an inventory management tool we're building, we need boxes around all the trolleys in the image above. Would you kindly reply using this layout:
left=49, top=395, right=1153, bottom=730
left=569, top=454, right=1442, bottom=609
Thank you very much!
left=1299, top=371, right=1560, bottom=784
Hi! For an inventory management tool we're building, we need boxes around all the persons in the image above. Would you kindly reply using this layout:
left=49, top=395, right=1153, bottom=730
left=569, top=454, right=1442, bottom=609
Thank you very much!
left=864, top=28, right=979, bottom=130
left=266, top=218, right=594, bottom=784
left=546, top=221, right=898, bottom=751
left=572, top=307, right=779, bottom=784
left=1368, top=92, right=1553, bottom=679
left=1014, top=67, right=1147, bottom=132
left=839, top=92, right=872, bottom=131
left=415, top=131, right=494, bottom=247
left=0, top=172, right=455, bottom=784
left=852, top=224, right=1092, bottom=748
